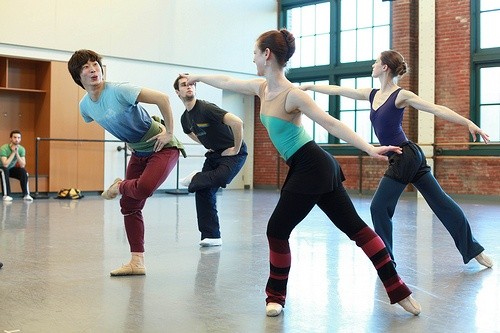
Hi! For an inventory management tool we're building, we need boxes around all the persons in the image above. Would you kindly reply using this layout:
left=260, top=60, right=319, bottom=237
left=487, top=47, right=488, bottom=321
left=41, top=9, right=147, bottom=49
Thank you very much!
left=67, top=49, right=188, bottom=277
left=297, top=51, right=494, bottom=269
left=0, top=129, right=33, bottom=201
left=179, top=30, right=422, bottom=316
left=174, top=73, right=249, bottom=247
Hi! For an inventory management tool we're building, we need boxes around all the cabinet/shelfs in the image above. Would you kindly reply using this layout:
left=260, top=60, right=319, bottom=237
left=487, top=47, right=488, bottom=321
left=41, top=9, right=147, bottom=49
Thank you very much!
left=0, top=55, right=106, bottom=192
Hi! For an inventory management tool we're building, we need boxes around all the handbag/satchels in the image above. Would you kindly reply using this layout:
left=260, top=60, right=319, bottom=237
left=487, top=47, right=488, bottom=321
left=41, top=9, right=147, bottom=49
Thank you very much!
left=53, top=188, right=84, bottom=199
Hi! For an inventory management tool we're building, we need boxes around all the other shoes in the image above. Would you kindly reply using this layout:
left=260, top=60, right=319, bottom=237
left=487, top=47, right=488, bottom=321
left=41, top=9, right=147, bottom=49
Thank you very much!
left=24, top=195, right=33, bottom=201
left=266, top=303, right=282, bottom=316
left=179, top=171, right=198, bottom=186
left=110, top=263, right=146, bottom=275
left=474, top=251, right=494, bottom=268
left=101, top=178, right=122, bottom=199
left=3, top=196, right=13, bottom=200
left=397, top=294, right=421, bottom=315
left=199, top=238, right=222, bottom=246
